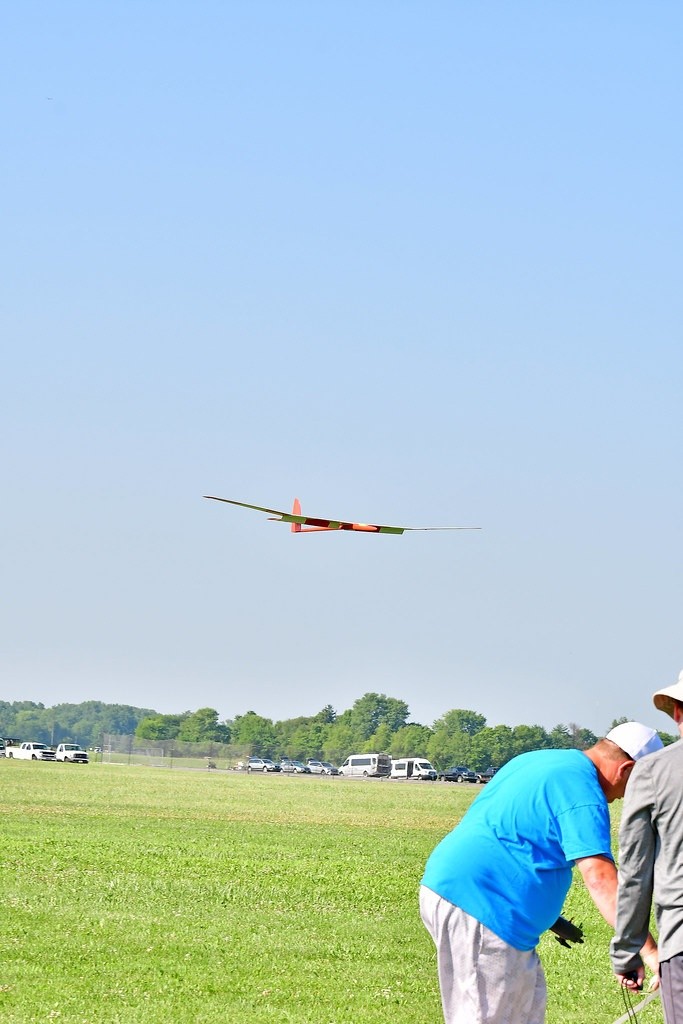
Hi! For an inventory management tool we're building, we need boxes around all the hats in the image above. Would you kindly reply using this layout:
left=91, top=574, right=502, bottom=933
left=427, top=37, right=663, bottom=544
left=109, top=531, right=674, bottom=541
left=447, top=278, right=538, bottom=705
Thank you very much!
left=606, top=721, right=664, bottom=761
left=653, top=668, right=683, bottom=722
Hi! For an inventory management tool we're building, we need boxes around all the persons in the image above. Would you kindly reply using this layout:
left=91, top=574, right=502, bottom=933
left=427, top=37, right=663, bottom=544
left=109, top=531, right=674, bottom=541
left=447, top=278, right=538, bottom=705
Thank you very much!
left=610, top=671, right=683, bottom=1024
left=418, top=721, right=664, bottom=1024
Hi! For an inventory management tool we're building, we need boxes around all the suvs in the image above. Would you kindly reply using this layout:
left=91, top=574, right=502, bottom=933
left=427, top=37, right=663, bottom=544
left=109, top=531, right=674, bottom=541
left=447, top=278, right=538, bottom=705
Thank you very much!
left=55, top=743, right=90, bottom=764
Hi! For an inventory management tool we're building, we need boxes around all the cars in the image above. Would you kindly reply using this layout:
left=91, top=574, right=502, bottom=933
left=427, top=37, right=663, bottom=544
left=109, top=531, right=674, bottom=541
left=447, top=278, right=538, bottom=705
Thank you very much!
left=437, top=765, right=478, bottom=784
left=0, top=737, right=21, bottom=757
left=475, top=766, right=500, bottom=785
left=305, top=760, right=339, bottom=775
left=280, top=759, right=311, bottom=774
left=247, top=758, right=281, bottom=773
left=94, top=748, right=102, bottom=753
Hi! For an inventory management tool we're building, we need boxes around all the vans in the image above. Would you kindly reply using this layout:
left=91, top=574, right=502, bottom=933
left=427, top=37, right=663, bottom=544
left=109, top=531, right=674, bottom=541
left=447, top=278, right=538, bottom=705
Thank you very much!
left=389, top=758, right=438, bottom=782
left=337, top=752, right=392, bottom=778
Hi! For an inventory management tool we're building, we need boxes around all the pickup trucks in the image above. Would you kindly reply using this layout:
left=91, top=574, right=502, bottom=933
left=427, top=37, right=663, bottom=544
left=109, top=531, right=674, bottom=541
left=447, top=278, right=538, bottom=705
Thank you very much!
left=5, top=742, right=56, bottom=761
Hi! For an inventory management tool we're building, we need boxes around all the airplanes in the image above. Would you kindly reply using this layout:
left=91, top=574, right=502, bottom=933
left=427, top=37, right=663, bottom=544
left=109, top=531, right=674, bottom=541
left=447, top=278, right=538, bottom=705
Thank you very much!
left=202, top=493, right=482, bottom=535
